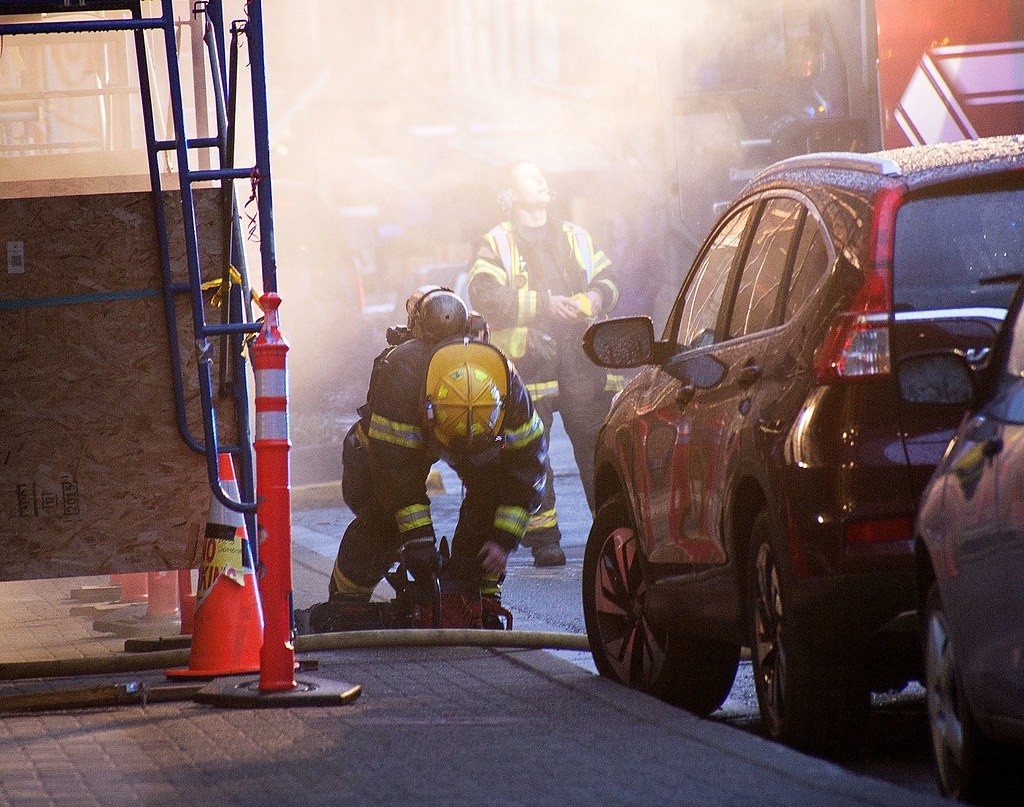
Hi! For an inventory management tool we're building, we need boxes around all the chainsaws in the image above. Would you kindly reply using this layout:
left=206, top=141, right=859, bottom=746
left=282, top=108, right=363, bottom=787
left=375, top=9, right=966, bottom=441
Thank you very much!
left=293, top=560, right=514, bottom=630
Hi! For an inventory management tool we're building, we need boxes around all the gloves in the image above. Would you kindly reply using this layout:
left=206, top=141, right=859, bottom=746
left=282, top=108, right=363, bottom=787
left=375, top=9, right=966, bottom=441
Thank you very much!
left=402, top=543, right=438, bottom=580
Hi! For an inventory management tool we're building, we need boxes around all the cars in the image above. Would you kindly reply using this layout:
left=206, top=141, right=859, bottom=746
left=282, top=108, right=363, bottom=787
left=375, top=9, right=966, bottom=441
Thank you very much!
left=913, top=284, right=1024, bottom=805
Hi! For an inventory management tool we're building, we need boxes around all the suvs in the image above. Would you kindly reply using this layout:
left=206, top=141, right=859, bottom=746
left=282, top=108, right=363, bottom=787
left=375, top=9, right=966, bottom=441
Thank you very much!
left=581, top=132, right=1023, bottom=760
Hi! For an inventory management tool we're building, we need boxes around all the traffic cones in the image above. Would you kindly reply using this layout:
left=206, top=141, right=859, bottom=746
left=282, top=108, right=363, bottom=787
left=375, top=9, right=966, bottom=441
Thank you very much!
left=165, top=453, right=300, bottom=680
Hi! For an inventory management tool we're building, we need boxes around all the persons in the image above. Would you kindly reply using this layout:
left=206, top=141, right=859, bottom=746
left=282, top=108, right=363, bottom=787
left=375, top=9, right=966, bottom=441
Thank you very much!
left=468, top=159, right=619, bottom=568
left=328, top=338, right=547, bottom=629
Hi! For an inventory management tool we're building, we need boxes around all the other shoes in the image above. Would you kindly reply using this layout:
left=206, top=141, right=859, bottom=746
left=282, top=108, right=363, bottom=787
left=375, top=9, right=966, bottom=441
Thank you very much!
left=478, top=572, right=507, bottom=629
left=531, top=543, right=566, bottom=567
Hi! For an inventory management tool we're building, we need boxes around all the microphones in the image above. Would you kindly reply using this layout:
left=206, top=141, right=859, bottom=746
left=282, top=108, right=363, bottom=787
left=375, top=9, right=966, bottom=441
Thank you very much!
left=548, top=190, right=557, bottom=195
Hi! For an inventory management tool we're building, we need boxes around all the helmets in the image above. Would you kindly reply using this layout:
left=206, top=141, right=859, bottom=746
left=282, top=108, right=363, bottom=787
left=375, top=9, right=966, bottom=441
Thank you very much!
left=421, top=340, right=511, bottom=455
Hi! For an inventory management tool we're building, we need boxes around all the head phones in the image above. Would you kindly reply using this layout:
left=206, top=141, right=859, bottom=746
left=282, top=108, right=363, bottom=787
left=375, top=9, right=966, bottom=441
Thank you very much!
left=496, top=191, right=513, bottom=211
